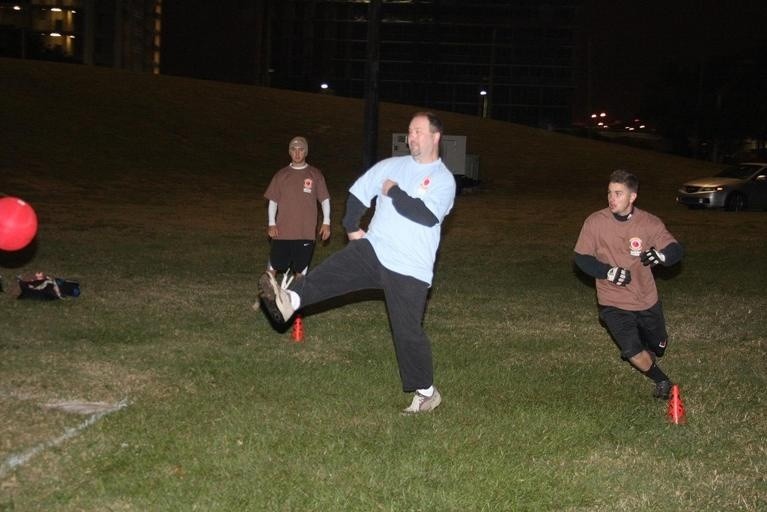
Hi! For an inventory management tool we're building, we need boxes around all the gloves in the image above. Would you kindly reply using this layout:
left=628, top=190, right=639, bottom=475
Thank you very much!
left=639, top=247, right=666, bottom=267
left=607, top=266, right=631, bottom=286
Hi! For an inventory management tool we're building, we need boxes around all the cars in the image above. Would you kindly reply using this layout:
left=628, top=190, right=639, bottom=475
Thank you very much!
left=674, top=161, right=767, bottom=212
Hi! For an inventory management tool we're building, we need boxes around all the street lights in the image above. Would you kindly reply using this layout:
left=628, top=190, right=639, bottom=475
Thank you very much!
left=480, top=89, right=488, bottom=118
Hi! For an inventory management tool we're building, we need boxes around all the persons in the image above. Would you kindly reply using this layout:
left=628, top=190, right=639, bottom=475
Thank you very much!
left=573, top=166, right=686, bottom=401
left=262, top=136, right=332, bottom=277
left=257, top=111, right=457, bottom=415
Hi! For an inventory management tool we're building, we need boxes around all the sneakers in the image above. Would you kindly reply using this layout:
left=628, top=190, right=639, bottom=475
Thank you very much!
left=401, top=385, right=441, bottom=417
left=656, top=338, right=667, bottom=357
left=653, top=381, right=672, bottom=399
left=258, top=271, right=294, bottom=324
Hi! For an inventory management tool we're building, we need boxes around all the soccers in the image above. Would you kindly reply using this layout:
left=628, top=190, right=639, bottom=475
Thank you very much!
left=0, top=197, right=38, bottom=250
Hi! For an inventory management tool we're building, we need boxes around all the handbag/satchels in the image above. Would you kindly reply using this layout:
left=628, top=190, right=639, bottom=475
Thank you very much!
left=16, top=274, right=65, bottom=300
